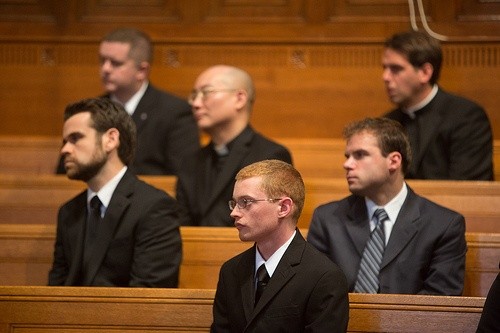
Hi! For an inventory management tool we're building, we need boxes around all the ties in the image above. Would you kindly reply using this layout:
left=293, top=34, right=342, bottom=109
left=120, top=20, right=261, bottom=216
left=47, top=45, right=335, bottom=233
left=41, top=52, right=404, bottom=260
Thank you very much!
left=254, top=264, right=270, bottom=306
left=352, top=208, right=390, bottom=294
left=80, top=195, right=102, bottom=277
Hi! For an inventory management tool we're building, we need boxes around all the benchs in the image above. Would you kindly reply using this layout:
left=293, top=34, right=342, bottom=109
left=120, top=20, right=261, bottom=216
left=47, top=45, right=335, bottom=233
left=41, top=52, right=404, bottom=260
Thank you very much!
left=0, top=135, right=500, bottom=333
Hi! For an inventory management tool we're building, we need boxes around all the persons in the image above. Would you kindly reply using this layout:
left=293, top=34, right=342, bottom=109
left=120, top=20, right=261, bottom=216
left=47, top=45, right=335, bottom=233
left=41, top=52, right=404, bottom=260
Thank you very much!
left=476, top=270, right=500, bottom=333
left=176, top=66, right=294, bottom=227
left=48, top=98, right=183, bottom=290
left=208, top=160, right=350, bottom=333
left=55, top=27, right=202, bottom=176
left=304, top=119, right=467, bottom=297
left=379, top=31, right=494, bottom=182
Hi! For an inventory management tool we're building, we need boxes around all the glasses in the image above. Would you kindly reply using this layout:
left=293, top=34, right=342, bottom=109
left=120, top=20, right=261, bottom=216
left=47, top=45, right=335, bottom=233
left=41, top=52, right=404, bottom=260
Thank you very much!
left=188, top=89, right=237, bottom=105
left=229, top=198, right=282, bottom=210
